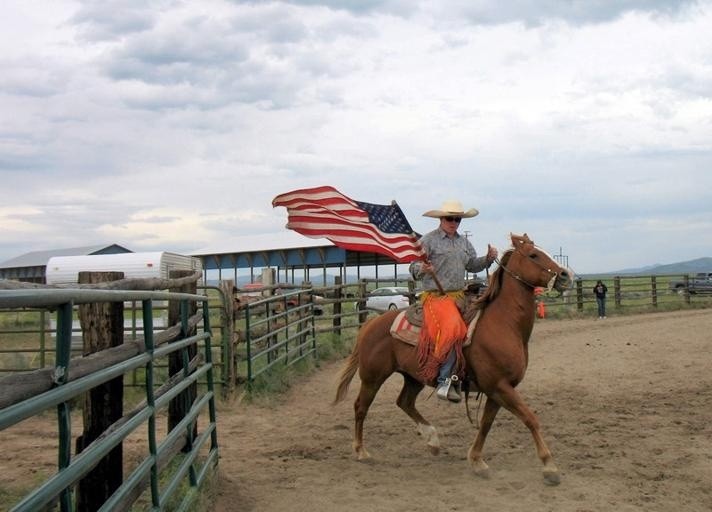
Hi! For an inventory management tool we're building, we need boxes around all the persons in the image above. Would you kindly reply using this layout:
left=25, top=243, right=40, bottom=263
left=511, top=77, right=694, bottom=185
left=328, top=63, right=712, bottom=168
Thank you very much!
left=408, top=200, right=499, bottom=403
left=593, top=280, right=607, bottom=319
left=468, top=273, right=483, bottom=281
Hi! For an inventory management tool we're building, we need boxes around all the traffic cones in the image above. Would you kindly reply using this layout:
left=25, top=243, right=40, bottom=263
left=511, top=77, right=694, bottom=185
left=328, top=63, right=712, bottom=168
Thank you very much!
left=540, top=303, right=544, bottom=318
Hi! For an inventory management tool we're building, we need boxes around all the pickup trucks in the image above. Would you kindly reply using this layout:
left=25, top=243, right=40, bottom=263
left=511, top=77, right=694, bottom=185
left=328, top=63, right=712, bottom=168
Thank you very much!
left=669, top=271, right=712, bottom=297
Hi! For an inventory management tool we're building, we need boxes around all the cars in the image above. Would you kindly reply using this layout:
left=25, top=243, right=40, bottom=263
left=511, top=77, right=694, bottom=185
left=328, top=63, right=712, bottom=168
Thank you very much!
left=353, top=287, right=423, bottom=312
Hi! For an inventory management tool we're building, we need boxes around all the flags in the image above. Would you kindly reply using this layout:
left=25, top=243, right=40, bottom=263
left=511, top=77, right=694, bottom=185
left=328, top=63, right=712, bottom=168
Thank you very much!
left=271, top=185, right=428, bottom=262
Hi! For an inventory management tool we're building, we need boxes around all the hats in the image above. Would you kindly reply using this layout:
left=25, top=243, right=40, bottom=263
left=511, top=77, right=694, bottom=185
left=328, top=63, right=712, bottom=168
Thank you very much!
left=423, top=199, right=479, bottom=218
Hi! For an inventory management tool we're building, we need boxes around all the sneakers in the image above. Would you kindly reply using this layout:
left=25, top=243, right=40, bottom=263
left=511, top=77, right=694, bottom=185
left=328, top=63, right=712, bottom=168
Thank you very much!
left=599, top=316, right=606, bottom=319
left=437, top=383, right=461, bottom=402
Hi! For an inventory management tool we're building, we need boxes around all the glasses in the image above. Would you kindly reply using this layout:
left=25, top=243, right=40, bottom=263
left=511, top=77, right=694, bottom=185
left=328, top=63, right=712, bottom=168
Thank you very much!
left=444, top=216, right=462, bottom=223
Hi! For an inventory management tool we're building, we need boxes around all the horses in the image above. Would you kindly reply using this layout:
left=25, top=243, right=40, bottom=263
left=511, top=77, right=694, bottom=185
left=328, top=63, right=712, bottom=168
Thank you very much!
left=324, top=233, right=573, bottom=487
